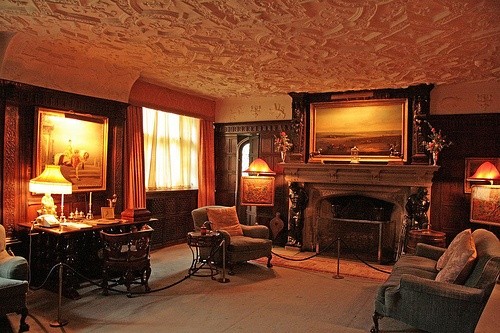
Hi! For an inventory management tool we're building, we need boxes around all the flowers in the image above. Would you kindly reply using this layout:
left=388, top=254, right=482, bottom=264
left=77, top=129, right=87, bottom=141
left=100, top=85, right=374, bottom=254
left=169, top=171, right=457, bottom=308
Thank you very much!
left=273, top=129, right=293, bottom=152
left=422, top=122, right=454, bottom=153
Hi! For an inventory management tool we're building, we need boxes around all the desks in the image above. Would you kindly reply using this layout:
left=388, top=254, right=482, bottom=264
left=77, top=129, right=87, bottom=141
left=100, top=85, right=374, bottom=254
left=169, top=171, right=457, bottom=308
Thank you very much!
left=20, top=213, right=158, bottom=300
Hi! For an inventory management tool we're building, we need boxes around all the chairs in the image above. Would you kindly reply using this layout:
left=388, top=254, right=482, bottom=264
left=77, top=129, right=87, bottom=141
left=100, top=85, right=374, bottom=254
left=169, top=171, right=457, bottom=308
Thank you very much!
left=97, top=224, right=151, bottom=295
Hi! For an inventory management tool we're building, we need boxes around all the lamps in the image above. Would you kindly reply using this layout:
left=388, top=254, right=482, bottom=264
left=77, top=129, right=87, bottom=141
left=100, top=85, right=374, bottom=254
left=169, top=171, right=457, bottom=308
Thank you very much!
left=29, top=165, right=72, bottom=218
left=243, top=158, right=276, bottom=176
left=472, top=161, right=500, bottom=185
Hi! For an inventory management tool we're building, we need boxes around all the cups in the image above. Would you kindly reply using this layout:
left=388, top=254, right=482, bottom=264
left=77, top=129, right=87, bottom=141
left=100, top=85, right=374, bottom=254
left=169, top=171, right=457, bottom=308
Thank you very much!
left=200, top=226, right=207, bottom=236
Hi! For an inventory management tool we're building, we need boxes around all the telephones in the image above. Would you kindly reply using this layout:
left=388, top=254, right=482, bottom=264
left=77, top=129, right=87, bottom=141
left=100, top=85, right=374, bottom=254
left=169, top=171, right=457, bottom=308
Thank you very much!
left=36, top=214, right=61, bottom=228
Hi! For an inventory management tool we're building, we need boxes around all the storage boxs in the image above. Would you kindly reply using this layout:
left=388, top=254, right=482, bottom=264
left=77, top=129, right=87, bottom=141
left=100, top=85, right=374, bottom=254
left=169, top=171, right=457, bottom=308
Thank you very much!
left=121, top=207, right=152, bottom=223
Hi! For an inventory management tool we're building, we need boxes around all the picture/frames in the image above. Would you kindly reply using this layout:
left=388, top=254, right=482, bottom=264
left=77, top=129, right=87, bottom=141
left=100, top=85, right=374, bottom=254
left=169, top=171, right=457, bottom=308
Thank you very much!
left=308, top=98, right=408, bottom=162
left=31, top=107, right=109, bottom=190
left=240, top=176, right=275, bottom=206
left=470, top=184, right=500, bottom=226
left=463, top=157, right=500, bottom=193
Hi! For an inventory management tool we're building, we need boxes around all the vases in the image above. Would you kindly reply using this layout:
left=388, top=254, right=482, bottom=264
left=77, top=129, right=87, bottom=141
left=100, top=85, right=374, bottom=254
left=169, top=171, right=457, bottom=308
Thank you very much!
left=431, top=151, right=439, bottom=166
left=281, top=151, right=287, bottom=163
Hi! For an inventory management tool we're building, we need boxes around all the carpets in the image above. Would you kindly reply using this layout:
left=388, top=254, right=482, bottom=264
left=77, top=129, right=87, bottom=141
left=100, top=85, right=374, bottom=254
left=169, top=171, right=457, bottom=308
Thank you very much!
left=251, top=253, right=391, bottom=281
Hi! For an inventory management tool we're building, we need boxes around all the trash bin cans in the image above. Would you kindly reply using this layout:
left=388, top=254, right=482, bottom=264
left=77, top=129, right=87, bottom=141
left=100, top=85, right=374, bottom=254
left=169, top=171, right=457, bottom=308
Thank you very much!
left=406, top=223, right=446, bottom=255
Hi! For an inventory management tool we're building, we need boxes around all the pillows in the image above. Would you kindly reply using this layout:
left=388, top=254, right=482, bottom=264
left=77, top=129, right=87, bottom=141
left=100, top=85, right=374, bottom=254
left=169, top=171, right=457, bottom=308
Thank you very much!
left=434, top=229, right=477, bottom=285
left=205, top=206, right=244, bottom=236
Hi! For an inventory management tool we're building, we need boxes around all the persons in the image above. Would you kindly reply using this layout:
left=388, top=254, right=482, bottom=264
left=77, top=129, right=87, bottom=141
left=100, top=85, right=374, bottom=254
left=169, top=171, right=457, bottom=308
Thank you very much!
left=405, top=187, right=431, bottom=231
left=288, top=182, right=309, bottom=248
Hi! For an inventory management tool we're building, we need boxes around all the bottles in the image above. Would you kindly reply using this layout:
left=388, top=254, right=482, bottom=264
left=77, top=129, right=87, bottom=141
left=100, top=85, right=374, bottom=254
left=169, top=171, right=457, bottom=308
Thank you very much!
left=351, top=146, right=359, bottom=163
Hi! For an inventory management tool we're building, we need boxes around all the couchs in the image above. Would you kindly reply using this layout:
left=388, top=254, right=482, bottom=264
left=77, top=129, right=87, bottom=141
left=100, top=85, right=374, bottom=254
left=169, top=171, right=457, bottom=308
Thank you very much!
left=370, top=228, right=500, bottom=333
left=187, top=206, right=274, bottom=275
left=0, top=224, right=31, bottom=333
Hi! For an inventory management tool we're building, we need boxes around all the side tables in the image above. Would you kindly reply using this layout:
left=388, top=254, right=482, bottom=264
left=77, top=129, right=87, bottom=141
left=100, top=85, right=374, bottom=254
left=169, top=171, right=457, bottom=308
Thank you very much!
left=185, top=231, right=223, bottom=279
